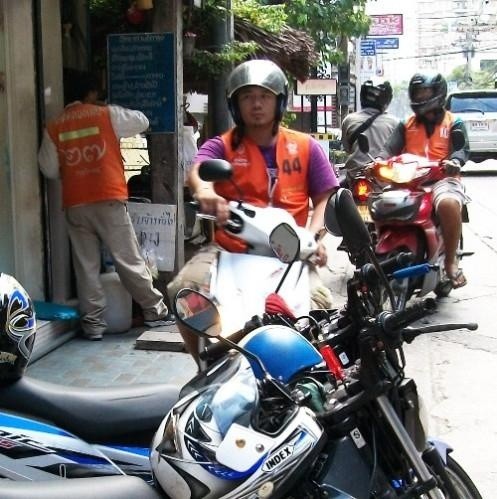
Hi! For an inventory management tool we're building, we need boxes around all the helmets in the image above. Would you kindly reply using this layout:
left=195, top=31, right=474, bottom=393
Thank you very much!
left=358, top=75, right=394, bottom=115
left=405, top=70, right=450, bottom=117
left=222, top=56, right=291, bottom=129
left=232, top=322, right=325, bottom=387
left=0, top=271, right=38, bottom=389
left=144, top=377, right=332, bottom=499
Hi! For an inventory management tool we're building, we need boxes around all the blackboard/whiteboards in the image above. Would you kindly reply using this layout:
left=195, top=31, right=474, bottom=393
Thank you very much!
left=107, top=31, right=178, bottom=135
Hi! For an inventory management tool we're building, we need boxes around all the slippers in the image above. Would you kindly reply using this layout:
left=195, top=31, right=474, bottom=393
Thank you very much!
left=444, top=267, right=468, bottom=290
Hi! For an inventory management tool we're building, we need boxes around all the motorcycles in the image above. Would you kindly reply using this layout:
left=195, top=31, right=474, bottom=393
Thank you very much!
left=352, top=134, right=474, bottom=331
left=0, top=188, right=483, bottom=499
left=335, top=158, right=385, bottom=272
left=181, top=158, right=336, bottom=376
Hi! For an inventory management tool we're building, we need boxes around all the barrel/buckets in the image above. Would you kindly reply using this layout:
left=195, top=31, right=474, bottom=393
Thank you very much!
left=97, top=265, right=132, bottom=333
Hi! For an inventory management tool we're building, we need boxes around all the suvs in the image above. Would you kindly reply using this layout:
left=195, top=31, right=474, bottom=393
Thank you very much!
left=447, top=89, right=497, bottom=167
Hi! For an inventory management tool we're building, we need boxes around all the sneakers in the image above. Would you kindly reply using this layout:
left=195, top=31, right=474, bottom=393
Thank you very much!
left=142, top=313, right=176, bottom=328
left=86, top=334, right=104, bottom=341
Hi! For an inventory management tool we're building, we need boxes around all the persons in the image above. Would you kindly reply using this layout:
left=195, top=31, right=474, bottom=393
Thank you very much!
left=37, top=71, right=176, bottom=341
left=165, top=58, right=344, bottom=374
left=363, top=72, right=467, bottom=290
left=338, top=77, right=399, bottom=246
